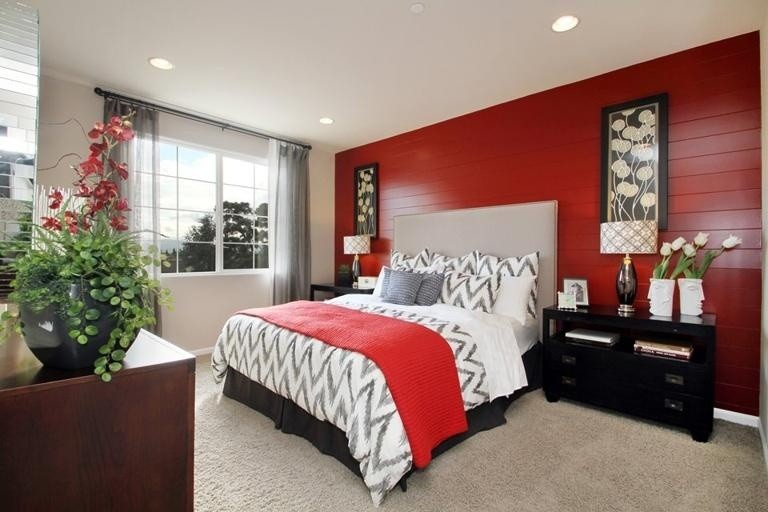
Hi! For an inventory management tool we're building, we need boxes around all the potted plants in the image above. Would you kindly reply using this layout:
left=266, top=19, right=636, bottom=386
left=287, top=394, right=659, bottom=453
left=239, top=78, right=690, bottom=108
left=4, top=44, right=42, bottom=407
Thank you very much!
left=338, top=264, right=352, bottom=286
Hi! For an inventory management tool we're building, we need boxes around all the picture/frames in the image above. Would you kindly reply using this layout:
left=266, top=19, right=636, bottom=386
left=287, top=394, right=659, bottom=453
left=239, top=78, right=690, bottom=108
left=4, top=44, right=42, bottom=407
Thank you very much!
left=562, top=277, right=590, bottom=308
left=599, top=91, right=669, bottom=232
left=353, top=161, right=380, bottom=242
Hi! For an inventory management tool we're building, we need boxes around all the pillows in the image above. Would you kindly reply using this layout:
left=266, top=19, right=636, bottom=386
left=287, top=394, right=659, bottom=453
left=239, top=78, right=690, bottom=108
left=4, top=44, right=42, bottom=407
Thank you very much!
left=373, top=247, right=540, bottom=326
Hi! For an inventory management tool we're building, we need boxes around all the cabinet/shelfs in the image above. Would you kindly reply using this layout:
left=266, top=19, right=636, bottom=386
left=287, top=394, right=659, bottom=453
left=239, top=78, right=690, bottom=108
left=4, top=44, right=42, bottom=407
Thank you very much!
left=542, top=303, right=717, bottom=443
left=0, top=303, right=197, bottom=512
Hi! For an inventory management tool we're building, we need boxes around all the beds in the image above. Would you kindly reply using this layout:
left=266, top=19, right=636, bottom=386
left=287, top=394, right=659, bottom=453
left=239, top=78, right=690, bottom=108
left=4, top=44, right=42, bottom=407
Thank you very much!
left=210, top=198, right=560, bottom=509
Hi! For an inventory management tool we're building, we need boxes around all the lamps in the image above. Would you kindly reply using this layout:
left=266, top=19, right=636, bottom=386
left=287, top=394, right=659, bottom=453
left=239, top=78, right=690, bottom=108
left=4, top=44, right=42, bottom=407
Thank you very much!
left=599, top=219, right=658, bottom=314
left=343, top=234, right=369, bottom=289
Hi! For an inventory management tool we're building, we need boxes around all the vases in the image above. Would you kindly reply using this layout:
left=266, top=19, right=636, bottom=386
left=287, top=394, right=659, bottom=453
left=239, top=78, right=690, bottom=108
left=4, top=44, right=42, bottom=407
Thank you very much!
left=18, top=282, right=145, bottom=372
left=680, top=314, right=704, bottom=325
left=677, top=278, right=705, bottom=316
left=648, top=315, right=672, bottom=322
left=646, top=277, right=676, bottom=317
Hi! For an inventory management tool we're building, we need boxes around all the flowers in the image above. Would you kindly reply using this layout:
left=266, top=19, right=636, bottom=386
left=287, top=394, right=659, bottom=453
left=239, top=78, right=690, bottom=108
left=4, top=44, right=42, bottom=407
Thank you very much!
left=652, top=230, right=711, bottom=279
left=0, top=109, right=175, bottom=386
left=681, top=233, right=743, bottom=277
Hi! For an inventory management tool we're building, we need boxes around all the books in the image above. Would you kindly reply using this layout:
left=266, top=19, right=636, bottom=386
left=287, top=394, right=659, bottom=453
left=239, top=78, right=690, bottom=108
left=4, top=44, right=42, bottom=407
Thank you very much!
left=633, top=339, right=694, bottom=363
left=564, top=328, right=620, bottom=348
